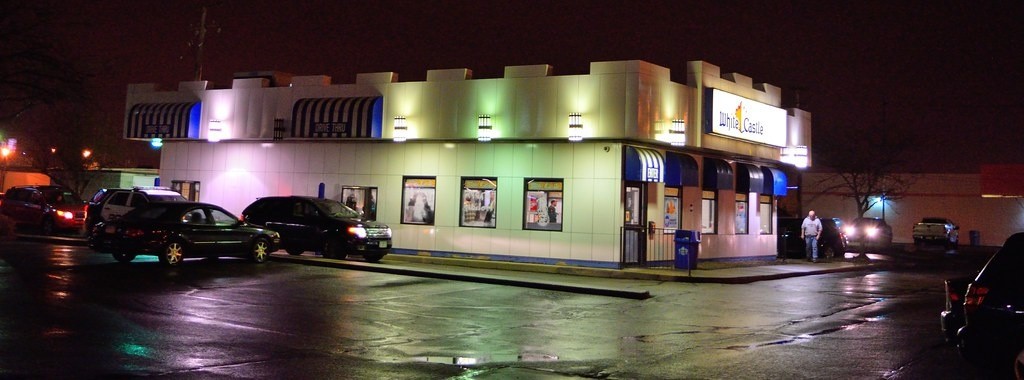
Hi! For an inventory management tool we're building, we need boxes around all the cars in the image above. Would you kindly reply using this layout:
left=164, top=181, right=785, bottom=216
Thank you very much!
left=777, top=216, right=846, bottom=261
left=846, top=218, right=893, bottom=250
left=87, top=200, right=281, bottom=263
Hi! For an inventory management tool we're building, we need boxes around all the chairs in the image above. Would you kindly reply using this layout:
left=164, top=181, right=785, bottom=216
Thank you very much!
left=192, top=212, right=202, bottom=224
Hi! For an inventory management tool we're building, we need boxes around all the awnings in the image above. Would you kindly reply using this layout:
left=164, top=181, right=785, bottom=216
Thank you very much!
left=126, top=102, right=201, bottom=138
left=625, top=146, right=787, bottom=195
left=292, top=96, right=384, bottom=138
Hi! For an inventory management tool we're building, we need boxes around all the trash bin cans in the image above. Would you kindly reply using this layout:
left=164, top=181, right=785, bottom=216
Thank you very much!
left=673, top=229, right=702, bottom=270
left=970, top=230, right=979, bottom=246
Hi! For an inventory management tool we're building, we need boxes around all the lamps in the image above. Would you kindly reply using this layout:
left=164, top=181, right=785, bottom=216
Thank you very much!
left=273, top=118, right=285, bottom=140
left=669, top=119, right=685, bottom=146
left=568, top=112, right=583, bottom=141
left=478, top=114, right=492, bottom=142
left=795, top=146, right=807, bottom=168
left=393, top=116, right=407, bottom=142
left=208, top=120, right=222, bottom=142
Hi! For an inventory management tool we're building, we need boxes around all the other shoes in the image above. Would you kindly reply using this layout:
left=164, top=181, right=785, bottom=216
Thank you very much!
left=807, top=257, right=817, bottom=262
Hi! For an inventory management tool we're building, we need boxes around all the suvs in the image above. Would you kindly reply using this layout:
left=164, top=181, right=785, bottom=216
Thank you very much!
left=2, top=185, right=86, bottom=236
left=86, top=186, right=189, bottom=234
left=241, top=195, right=392, bottom=261
left=939, top=231, right=1023, bottom=379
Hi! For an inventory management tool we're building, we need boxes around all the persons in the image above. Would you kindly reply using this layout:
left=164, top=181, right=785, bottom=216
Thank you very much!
left=52, top=193, right=64, bottom=204
left=548, top=200, right=558, bottom=222
left=801, top=211, right=823, bottom=262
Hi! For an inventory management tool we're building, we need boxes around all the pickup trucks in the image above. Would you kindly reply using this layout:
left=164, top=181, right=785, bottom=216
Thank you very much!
left=912, top=217, right=960, bottom=244
